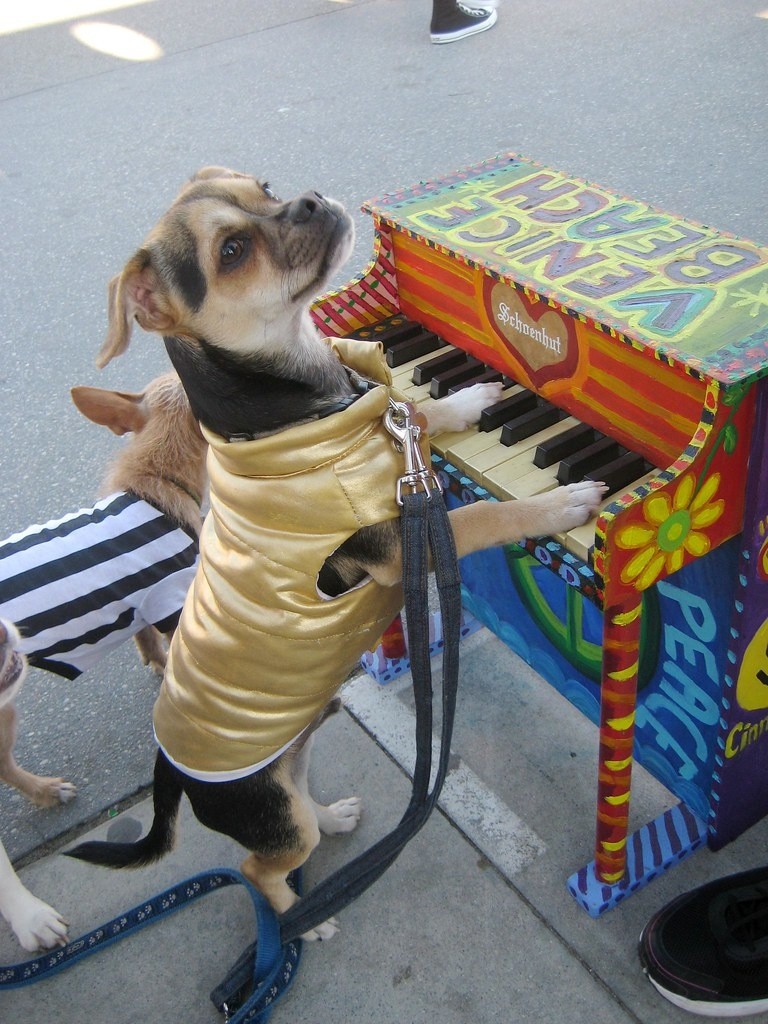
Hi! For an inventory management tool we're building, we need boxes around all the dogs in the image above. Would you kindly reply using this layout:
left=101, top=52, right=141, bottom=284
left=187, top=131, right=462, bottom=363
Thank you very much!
left=0, top=370, right=209, bottom=954
left=62, top=164, right=615, bottom=941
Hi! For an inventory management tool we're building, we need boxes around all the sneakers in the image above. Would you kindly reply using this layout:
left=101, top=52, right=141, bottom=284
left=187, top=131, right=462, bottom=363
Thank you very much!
left=430, top=0, right=498, bottom=44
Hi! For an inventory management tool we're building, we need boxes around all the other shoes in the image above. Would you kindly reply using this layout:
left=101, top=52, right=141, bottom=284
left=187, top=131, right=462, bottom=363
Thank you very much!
left=638, top=864, right=768, bottom=1016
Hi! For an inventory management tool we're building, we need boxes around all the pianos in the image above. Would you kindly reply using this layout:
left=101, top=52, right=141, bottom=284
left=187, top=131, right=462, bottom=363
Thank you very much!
left=308, top=151, right=768, bottom=920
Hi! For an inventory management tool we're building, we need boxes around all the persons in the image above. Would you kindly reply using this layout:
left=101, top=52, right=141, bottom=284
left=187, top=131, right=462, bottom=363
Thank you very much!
left=430, top=0, right=497, bottom=43
left=639, top=866, right=768, bottom=1016
